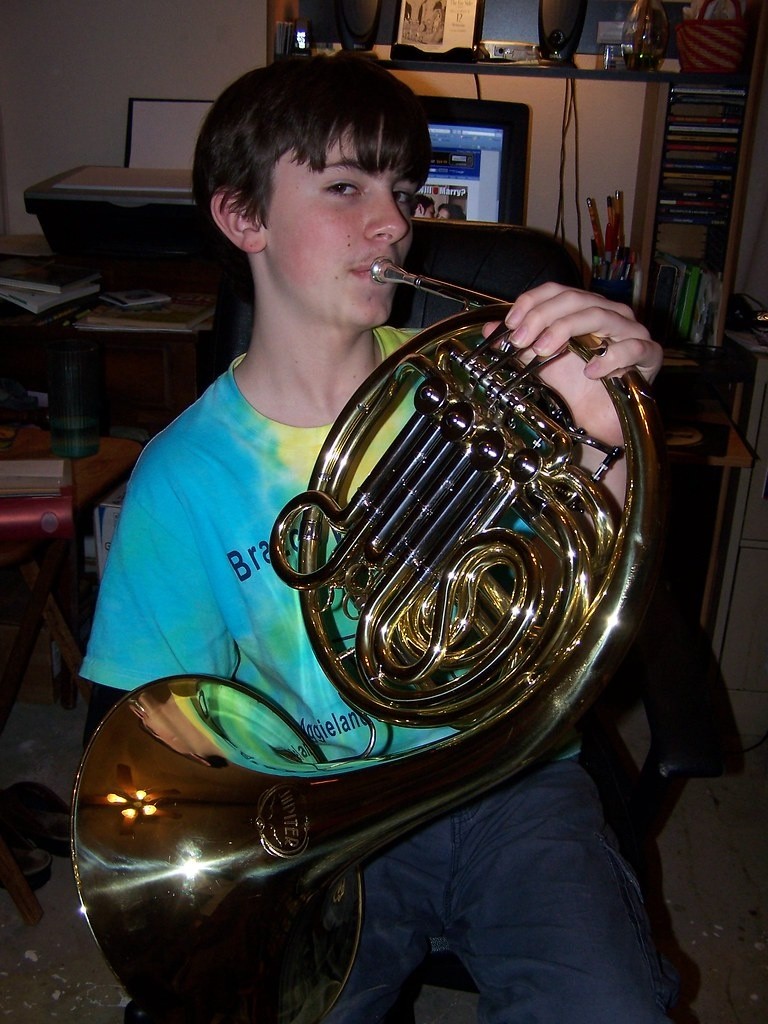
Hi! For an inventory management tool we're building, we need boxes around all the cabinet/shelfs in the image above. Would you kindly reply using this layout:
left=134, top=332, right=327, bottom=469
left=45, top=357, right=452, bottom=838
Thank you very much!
left=261, top=0, right=768, bottom=636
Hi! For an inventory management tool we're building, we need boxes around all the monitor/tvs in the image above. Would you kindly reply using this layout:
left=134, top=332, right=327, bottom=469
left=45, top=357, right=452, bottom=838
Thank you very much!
left=408, top=95, right=530, bottom=226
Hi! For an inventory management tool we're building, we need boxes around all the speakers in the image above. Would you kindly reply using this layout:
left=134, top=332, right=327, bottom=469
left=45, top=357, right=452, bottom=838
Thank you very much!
left=336, top=0, right=382, bottom=59
left=538, top=0, right=588, bottom=68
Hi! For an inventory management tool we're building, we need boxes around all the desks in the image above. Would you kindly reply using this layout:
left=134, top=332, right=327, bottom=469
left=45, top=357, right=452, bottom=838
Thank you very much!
left=0, top=233, right=223, bottom=433
left=0, top=425, right=143, bottom=925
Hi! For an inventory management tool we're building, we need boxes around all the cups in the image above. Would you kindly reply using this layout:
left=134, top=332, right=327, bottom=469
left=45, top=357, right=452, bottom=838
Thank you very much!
left=603, top=45, right=624, bottom=70
left=47, top=341, right=100, bottom=459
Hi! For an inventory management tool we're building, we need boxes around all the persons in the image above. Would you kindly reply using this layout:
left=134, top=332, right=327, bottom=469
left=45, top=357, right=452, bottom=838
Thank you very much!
left=436, top=203, right=466, bottom=220
left=75, top=52, right=684, bottom=1024
left=409, top=193, right=435, bottom=218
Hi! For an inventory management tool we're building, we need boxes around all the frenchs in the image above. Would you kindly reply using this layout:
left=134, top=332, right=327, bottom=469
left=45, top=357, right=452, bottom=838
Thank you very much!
left=67, top=253, right=659, bottom=1023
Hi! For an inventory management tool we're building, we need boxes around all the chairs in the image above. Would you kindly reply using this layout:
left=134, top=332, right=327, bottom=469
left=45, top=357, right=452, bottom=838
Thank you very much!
left=89, top=221, right=734, bottom=1024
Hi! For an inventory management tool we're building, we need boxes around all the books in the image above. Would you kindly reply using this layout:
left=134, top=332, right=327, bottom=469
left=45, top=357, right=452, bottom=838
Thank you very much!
left=1, top=254, right=219, bottom=334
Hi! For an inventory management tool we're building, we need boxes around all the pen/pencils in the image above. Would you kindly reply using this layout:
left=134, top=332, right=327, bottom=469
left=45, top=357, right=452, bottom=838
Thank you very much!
left=586, top=190, right=631, bottom=280
left=62, top=309, right=92, bottom=327
left=36, top=306, right=80, bottom=327
